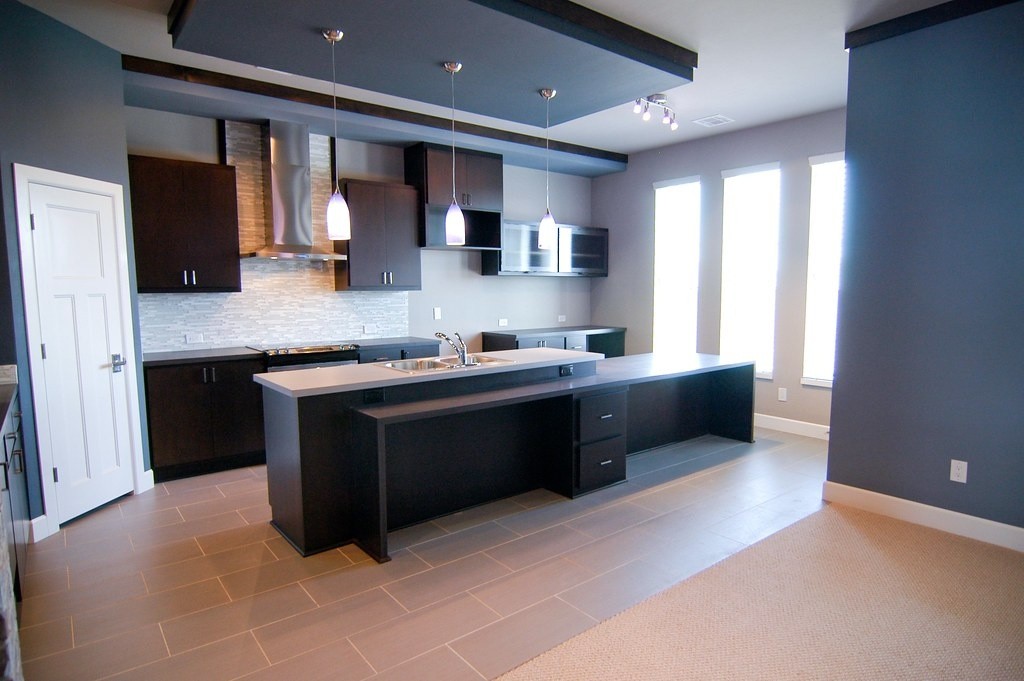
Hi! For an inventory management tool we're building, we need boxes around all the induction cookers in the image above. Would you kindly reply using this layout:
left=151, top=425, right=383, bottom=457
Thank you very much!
left=245, top=341, right=355, bottom=366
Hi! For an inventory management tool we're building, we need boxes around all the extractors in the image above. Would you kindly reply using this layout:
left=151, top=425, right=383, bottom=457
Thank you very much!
left=240, top=120, right=348, bottom=262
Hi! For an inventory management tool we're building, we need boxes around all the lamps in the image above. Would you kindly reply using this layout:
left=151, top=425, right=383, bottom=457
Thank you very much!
left=538, top=88, right=559, bottom=250
left=322, top=28, right=352, bottom=242
left=634, top=93, right=679, bottom=131
left=442, top=62, right=468, bottom=246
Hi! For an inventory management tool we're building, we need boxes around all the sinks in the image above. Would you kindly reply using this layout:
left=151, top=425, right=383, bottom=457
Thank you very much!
left=385, top=359, right=449, bottom=372
left=438, top=355, right=514, bottom=368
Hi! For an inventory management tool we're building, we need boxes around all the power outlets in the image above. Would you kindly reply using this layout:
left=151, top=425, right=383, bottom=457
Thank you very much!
left=499, top=319, right=508, bottom=326
left=433, top=307, right=442, bottom=320
left=950, top=460, right=968, bottom=484
left=558, top=315, right=567, bottom=322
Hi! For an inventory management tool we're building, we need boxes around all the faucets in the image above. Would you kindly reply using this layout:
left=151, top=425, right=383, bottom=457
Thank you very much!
left=435, top=331, right=467, bottom=365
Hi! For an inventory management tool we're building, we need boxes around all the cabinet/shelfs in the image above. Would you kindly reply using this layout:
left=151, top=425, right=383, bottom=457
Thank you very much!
left=481, top=325, right=627, bottom=358
left=0, top=385, right=48, bottom=631
left=127, top=154, right=244, bottom=293
left=348, top=348, right=756, bottom=566
left=481, top=218, right=609, bottom=279
left=139, top=334, right=442, bottom=483
left=403, top=142, right=503, bottom=251
left=333, top=178, right=423, bottom=291
